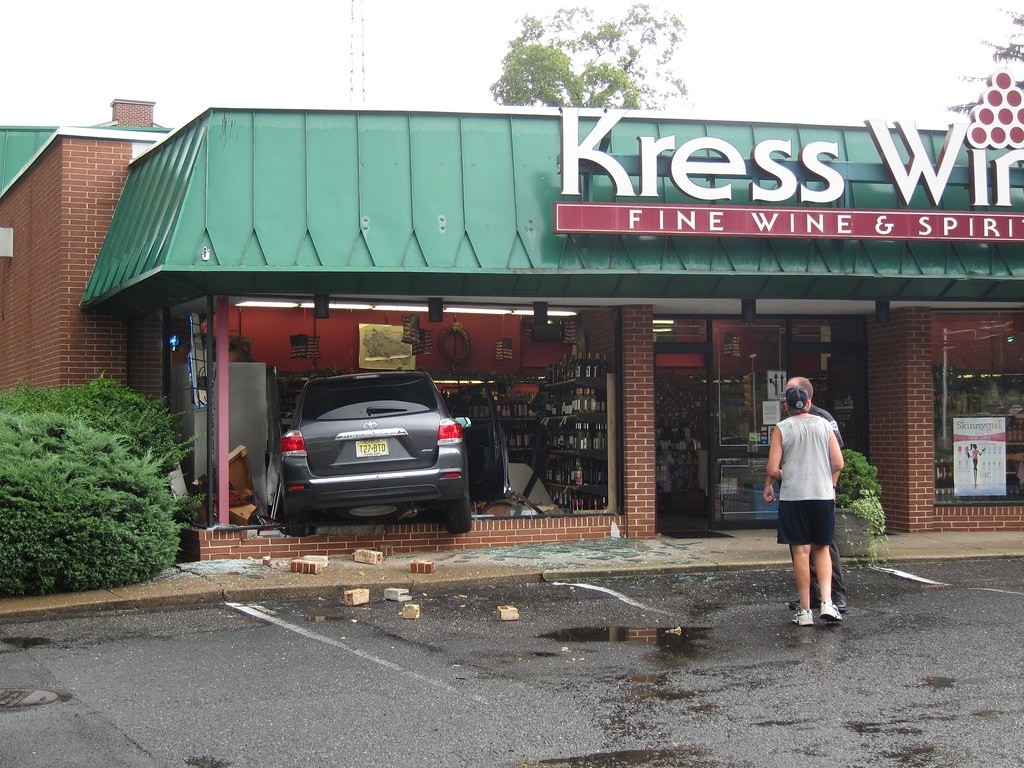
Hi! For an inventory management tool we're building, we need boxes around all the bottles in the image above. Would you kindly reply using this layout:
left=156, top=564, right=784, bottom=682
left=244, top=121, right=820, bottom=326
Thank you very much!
left=547, top=430, right=564, bottom=449
left=550, top=490, right=608, bottom=510
left=439, top=390, right=490, bottom=417
left=960, top=482, right=1024, bottom=501
left=838, top=414, right=856, bottom=432
left=1006, top=425, right=1024, bottom=443
left=939, top=426, right=952, bottom=453
left=546, top=387, right=606, bottom=416
left=506, top=423, right=535, bottom=448
left=933, top=386, right=981, bottom=413
left=546, top=345, right=608, bottom=384
left=491, top=390, right=538, bottom=417
left=568, top=423, right=607, bottom=450
left=999, top=378, right=1021, bottom=395
left=936, top=466, right=954, bottom=504
left=544, top=458, right=608, bottom=484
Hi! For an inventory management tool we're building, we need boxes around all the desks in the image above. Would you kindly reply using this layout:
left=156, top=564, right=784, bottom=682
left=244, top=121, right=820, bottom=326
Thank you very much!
left=466, top=371, right=618, bottom=515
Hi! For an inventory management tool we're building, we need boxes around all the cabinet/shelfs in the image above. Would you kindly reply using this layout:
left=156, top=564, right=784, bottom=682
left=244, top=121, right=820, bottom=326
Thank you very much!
left=934, top=405, right=1024, bottom=489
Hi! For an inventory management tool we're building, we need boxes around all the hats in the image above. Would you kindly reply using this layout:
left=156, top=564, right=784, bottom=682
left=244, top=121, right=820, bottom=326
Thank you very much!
left=786, top=387, right=808, bottom=409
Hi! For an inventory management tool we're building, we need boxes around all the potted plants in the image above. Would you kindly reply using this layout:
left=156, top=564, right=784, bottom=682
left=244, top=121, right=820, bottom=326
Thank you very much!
left=228, top=337, right=252, bottom=362
left=833, top=449, right=895, bottom=572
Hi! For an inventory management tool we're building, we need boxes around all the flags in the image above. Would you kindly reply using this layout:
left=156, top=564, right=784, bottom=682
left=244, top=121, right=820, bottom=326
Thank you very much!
left=563, top=320, right=576, bottom=344
left=723, top=334, right=740, bottom=356
left=412, top=328, right=434, bottom=356
left=290, top=334, right=321, bottom=358
left=496, top=337, right=513, bottom=361
left=400, top=314, right=421, bottom=344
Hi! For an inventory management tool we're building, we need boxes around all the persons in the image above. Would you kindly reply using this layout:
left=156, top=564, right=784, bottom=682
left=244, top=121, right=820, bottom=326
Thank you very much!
left=767, top=385, right=844, bottom=625
left=764, top=377, right=846, bottom=611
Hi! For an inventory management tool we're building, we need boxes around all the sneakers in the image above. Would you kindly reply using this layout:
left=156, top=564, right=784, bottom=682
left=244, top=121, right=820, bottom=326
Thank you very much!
left=832, top=596, right=847, bottom=612
left=789, top=599, right=821, bottom=610
left=820, top=601, right=843, bottom=622
left=792, top=610, right=814, bottom=626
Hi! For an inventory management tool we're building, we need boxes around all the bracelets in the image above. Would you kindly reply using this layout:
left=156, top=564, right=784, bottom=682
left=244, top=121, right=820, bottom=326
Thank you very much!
left=833, top=485, right=836, bottom=489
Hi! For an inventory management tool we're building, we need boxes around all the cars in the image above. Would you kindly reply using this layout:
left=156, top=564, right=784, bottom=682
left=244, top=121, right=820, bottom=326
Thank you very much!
left=279, top=371, right=512, bottom=537
